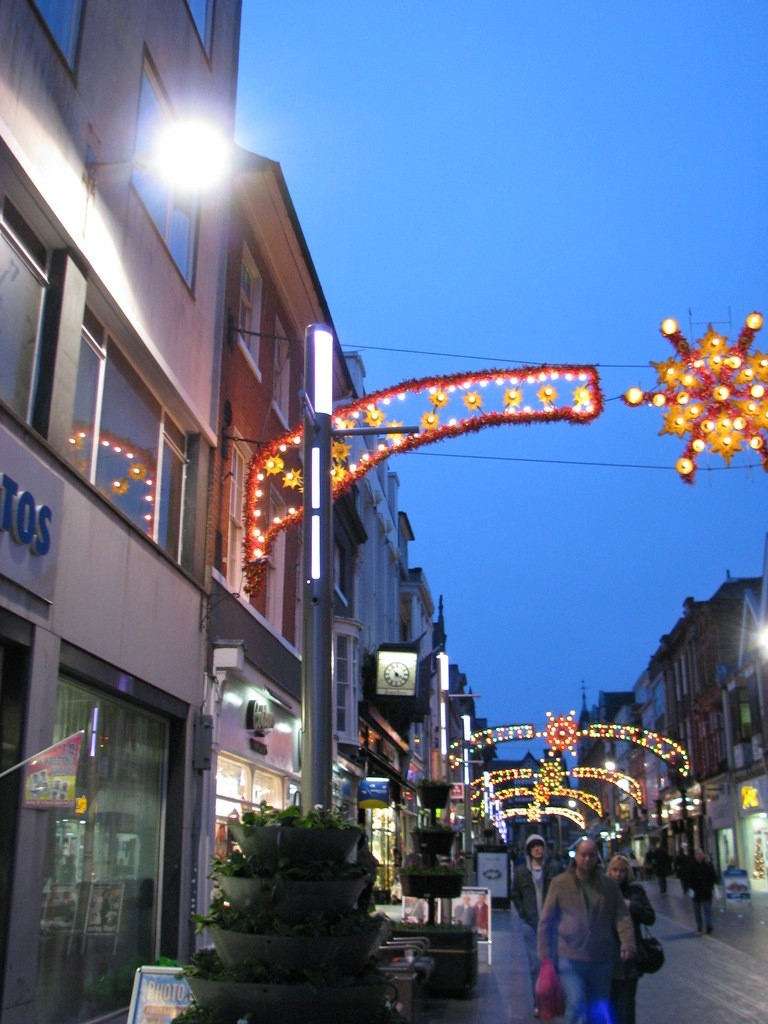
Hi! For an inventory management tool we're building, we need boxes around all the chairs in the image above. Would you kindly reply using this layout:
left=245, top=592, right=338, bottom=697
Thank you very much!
left=378, top=936, right=436, bottom=1024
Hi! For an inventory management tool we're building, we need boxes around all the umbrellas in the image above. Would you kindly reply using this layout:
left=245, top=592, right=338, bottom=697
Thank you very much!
left=620, top=847, right=635, bottom=853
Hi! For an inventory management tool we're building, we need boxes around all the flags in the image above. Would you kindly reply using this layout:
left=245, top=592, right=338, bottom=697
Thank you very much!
left=22, top=734, right=82, bottom=809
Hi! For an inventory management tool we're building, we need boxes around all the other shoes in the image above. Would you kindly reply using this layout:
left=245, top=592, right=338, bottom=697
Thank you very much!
left=698, top=928, right=701, bottom=932
left=706, top=925, right=713, bottom=935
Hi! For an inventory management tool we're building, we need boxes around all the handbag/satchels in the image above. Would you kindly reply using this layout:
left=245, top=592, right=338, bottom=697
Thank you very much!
left=532, top=955, right=566, bottom=1021
left=638, top=935, right=665, bottom=973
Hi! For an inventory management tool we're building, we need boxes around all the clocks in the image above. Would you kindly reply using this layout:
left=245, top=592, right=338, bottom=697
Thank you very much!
left=384, top=662, right=409, bottom=688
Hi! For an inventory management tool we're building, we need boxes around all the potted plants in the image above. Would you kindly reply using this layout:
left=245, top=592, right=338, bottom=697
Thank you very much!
left=397, top=861, right=471, bottom=901
left=393, top=923, right=479, bottom=1002
left=415, top=778, right=455, bottom=809
left=409, top=822, right=458, bottom=857
left=167, top=803, right=398, bottom=1024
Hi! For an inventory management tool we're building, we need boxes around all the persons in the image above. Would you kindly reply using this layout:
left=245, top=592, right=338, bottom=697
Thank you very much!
left=510, top=833, right=559, bottom=1019
left=628, top=852, right=641, bottom=879
left=688, top=849, right=722, bottom=934
left=536, top=839, right=637, bottom=1024
left=604, top=855, right=656, bottom=1024
left=652, top=841, right=693, bottom=894
